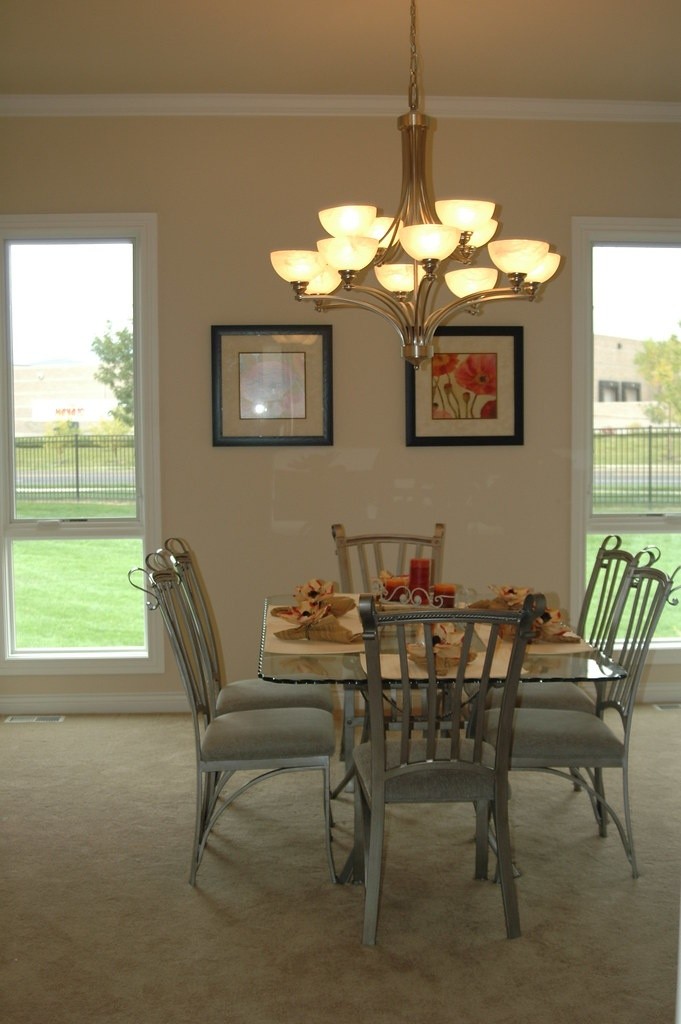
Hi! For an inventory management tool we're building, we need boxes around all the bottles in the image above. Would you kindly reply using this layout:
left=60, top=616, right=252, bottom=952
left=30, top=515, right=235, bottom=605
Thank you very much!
left=409, top=559, right=429, bottom=603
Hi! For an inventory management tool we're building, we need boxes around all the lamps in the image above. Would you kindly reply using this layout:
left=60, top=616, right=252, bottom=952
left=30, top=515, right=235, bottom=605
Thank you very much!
left=269, top=0, right=561, bottom=371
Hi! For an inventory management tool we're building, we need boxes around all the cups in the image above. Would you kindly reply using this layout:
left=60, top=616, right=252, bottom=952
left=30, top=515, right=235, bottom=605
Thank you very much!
left=384, top=578, right=407, bottom=602
left=432, top=584, right=458, bottom=609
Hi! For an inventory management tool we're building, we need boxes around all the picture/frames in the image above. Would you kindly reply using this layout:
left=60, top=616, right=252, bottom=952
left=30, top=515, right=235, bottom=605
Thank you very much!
left=211, top=325, right=334, bottom=447
left=404, top=326, right=524, bottom=446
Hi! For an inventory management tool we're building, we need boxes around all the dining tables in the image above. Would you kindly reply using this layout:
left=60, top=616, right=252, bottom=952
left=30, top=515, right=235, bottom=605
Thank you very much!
left=258, top=594, right=630, bottom=881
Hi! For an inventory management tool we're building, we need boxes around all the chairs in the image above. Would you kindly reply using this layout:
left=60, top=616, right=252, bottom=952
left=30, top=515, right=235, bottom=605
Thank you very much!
left=352, top=593, right=552, bottom=948
left=474, top=550, right=681, bottom=880
left=128, top=555, right=342, bottom=888
left=465, top=534, right=663, bottom=827
left=330, top=522, right=470, bottom=802
left=162, top=537, right=340, bottom=840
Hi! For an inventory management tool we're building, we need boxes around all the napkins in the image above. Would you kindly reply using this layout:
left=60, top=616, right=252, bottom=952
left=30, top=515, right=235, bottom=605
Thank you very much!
left=359, top=648, right=530, bottom=678
left=457, top=602, right=595, bottom=654
left=263, top=605, right=365, bottom=653
